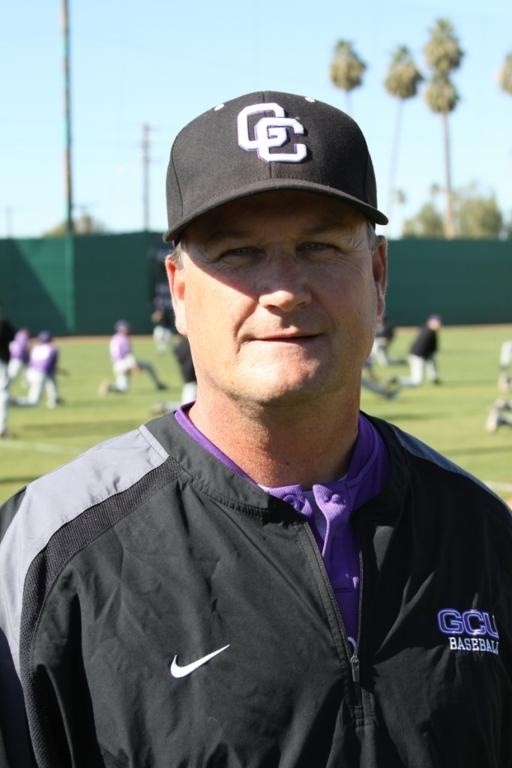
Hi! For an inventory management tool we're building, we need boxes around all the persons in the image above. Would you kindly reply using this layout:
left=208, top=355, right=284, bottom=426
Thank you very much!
left=395, top=314, right=442, bottom=387
left=7, top=332, right=66, bottom=410
left=362, top=357, right=402, bottom=402
left=486, top=392, right=512, bottom=432
left=3, top=328, right=39, bottom=395
left=370, top=310, right=406, bottom=369
left=151, top=291, right=175, bottom=360
left=497, top=343, right=512, bottom=394
left=139, top=337, right=198, bottom=417
left=1, top=89, right=512, bottom=768
left=99, top=319, right=170, bottom=396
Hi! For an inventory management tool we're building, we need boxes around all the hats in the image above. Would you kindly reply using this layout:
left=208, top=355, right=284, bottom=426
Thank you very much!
left=160, top=91, right=386, bottom=246
